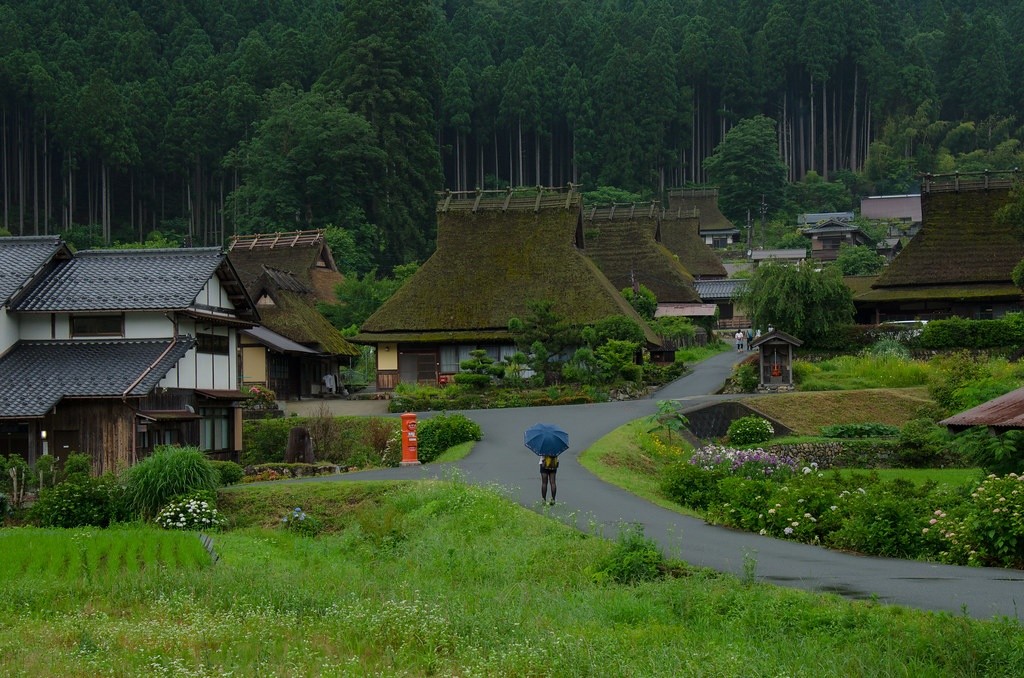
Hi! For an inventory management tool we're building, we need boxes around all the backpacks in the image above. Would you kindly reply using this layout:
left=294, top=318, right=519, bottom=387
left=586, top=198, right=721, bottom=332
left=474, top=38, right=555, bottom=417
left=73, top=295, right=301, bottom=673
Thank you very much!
left=545, top=455, right=557, bottom=470
left=737, top=336, right=741, bottom=340
left=748, top=334, right=753, bottom=341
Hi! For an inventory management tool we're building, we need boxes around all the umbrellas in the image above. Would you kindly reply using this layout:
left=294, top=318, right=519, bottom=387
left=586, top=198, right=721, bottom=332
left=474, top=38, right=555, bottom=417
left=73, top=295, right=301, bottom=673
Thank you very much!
left=524, top=423, right=570, bottom=456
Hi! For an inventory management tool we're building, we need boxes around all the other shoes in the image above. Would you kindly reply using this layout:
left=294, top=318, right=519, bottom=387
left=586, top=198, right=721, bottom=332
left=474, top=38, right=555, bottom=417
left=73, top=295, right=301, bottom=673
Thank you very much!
left=746, top=349, right=752, bottom=352
left=737, top=348, right=743, bottom=353
left=550, top=501, right=555, bottom=505
left=541, top=499, right=546, bottom=506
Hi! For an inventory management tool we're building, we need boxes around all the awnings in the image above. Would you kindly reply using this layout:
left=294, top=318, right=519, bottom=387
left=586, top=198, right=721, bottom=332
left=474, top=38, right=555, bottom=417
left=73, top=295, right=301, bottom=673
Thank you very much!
left=136, top=410, right=204, bottom=421
left=195, top=390, right=253, bottom=402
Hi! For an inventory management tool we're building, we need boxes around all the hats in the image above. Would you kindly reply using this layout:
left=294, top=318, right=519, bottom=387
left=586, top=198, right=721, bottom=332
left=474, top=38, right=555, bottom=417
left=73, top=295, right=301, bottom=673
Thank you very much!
left=767, top=324, right=772, bottom=328
left=737, top=330, right=741, bottom=333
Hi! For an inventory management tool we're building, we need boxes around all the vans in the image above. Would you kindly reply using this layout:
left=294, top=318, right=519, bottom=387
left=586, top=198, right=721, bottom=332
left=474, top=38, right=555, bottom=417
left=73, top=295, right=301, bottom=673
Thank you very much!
left=864, top=322, right=930, bottom=343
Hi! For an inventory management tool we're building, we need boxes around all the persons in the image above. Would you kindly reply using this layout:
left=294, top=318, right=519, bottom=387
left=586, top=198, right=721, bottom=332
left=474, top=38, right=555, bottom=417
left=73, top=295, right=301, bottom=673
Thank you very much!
left=745, top=325, right=754, bottom=350
left=768, top=324, right=774, bottom=332
left=539, top=455, right=559, bottom=505
left=735, top=329, right=744, bottom=352
left=755, top=328, right=761, bottom=351
